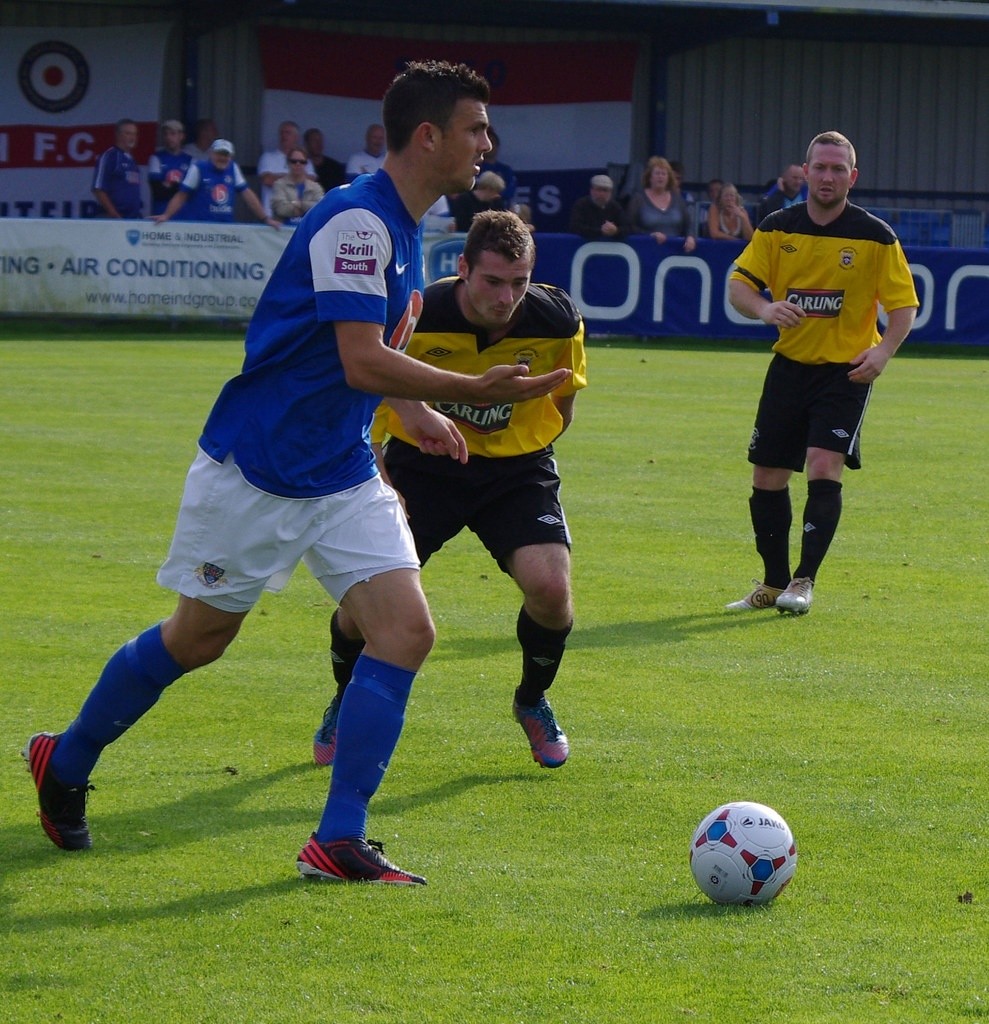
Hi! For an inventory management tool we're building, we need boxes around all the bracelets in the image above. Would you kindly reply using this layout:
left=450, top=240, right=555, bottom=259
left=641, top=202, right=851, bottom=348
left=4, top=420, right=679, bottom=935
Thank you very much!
left=262, top=216, right=267, bottom=223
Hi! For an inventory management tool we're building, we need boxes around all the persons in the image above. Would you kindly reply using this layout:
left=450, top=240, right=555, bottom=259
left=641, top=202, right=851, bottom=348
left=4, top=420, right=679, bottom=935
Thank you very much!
left=258, top=120, right=317, bottom=217
left=345, top=123, right=387, bottom=183
left=271, top=147, right=324, bottom=227
left=91, top=118, right=141, bottom=221
left=28, top=60, right=573, bottom=886
left=626, top=154, right=696, bottom=252
left=568, top=175, right=628, bottom=238
left=723, top=133, right=919, bottom=618
left=704, top=179, right=754, bottom=243
left=148, top=119, right=195, bottom=213
left=757, top=163, right=805, bottom=224
left=146, top=139, right=283, bottom=231
left=303, top=129, right=346, bottom=193
left=184, top=119, right=219, bottom=160
left=422, top=127, right=535, bottom=233
left=314, top=209, right=586, bottom=769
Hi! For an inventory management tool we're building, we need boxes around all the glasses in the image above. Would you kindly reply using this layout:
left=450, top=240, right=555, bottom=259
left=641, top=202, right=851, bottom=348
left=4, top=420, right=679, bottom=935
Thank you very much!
left=285, top=158, right=307, bottom=164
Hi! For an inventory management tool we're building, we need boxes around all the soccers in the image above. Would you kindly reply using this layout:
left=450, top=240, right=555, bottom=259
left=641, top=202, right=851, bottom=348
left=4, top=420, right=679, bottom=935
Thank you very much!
left=688, top=801, right=798, bottom=903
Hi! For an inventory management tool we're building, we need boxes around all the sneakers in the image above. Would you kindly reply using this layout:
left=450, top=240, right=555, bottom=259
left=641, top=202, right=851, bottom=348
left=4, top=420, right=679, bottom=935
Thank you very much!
left=725, top=577, right=785, bottom=613
left=775, top=575, right=813, bottom=616
left=295, top=831, right=428, bottom=886
left=20, top=730, right=93, bottom=851
left=313, top=698, right=343, bottom=768
left=512, top=685, right=570, bottom=768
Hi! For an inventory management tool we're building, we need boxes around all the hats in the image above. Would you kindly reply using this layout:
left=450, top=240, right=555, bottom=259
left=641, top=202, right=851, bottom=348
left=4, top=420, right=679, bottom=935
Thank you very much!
left=160, top=118, right=184, bottom=131
left=211, top=139, right=233, bottom=155
left=590, top=174, right=612, bottom=189
